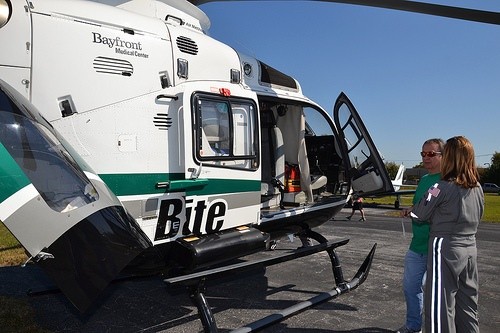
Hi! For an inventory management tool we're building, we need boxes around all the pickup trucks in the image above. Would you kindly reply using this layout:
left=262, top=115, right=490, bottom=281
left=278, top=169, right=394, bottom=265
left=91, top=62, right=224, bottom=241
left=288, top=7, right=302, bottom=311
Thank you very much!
left=482, top=183, right=500, bottom=193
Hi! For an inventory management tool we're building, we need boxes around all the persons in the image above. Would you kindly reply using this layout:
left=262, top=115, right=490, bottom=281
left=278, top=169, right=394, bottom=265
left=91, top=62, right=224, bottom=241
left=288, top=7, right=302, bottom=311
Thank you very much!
left=345, top=198, right=366, bottom=222
left=408, top=135, right=484, bottom=333
left=399, top=139, right=445, bottom=333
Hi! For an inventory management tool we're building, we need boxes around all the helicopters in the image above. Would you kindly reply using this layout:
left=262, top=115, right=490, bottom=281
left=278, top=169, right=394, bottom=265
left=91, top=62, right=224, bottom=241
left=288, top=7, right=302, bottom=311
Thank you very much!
left=0, top=1, right=500, bottom=333
left=347, top=164, right=417, bottom=210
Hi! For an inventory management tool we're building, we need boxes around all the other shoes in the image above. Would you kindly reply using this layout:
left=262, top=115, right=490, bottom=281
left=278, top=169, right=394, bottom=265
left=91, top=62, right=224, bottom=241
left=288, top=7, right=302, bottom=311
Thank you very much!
left=346, top=215, right=351, bottom=220
left=395, top=325, right=422, bottom=333
left=359, top=217, right=366, bottom=222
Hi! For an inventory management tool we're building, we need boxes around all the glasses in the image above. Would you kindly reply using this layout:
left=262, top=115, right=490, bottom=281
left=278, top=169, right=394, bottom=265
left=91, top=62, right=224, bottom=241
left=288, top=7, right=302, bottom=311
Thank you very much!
left=421, top=151, right=441, bottom=157
